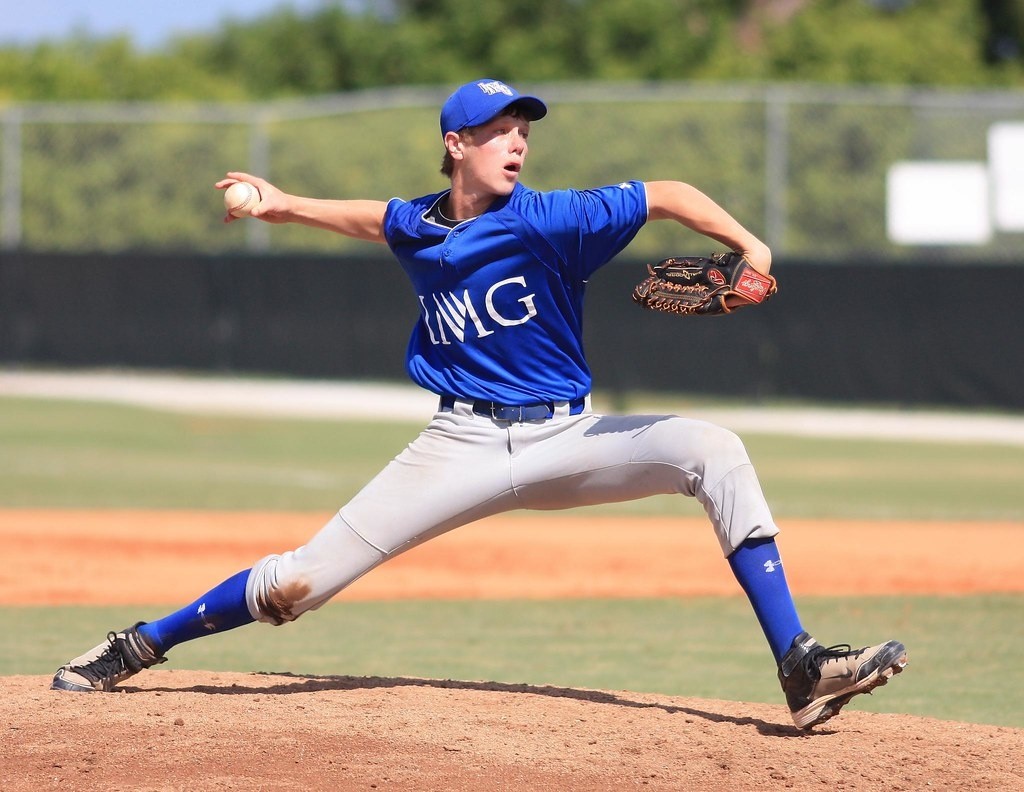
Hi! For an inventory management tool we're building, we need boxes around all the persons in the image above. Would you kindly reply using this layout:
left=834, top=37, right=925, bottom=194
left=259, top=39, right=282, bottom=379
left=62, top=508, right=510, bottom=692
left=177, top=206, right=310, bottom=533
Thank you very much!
left=54, top=79, right=907, bottom=729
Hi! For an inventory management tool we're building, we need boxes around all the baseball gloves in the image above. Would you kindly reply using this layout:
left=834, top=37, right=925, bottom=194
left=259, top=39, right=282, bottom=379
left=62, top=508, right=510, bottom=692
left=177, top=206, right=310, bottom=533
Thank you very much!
left=631, top=254, right=776, bottom=316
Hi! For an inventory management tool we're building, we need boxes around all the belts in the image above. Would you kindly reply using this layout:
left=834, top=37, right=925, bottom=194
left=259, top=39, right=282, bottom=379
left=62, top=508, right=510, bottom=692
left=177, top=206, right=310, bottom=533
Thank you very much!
left=440, top=395, right=584, bottom=422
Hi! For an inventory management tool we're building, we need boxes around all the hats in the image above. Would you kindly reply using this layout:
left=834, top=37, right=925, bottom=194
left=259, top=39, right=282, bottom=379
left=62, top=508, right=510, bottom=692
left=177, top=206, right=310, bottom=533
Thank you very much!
left=439, top=78, right=547, bottom=133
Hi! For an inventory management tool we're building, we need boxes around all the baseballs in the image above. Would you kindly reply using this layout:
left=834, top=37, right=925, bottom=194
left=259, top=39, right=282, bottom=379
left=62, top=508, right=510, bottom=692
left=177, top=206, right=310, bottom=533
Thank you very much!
left=223, top=182, right=261, bottom=217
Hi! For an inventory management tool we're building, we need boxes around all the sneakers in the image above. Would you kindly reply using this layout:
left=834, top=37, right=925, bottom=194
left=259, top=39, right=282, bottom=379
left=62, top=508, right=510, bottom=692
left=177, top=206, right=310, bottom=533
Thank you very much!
left=776, top=631, right=907, bottom=730
left=50, top=621, right=167, bottom=693
